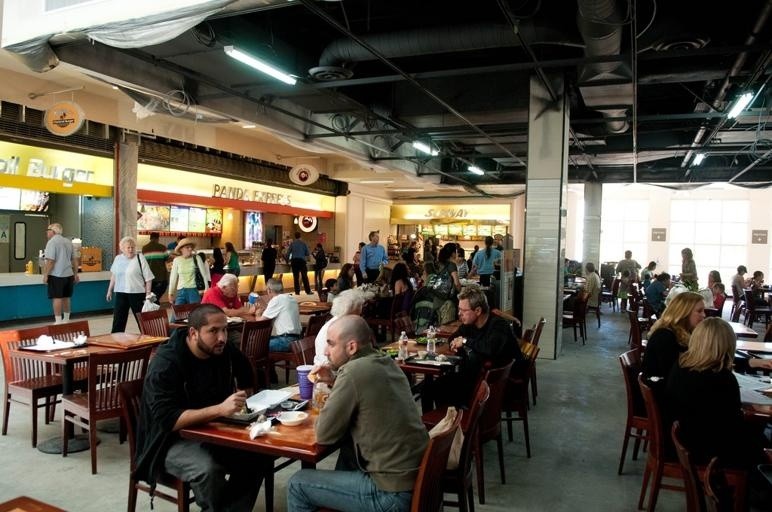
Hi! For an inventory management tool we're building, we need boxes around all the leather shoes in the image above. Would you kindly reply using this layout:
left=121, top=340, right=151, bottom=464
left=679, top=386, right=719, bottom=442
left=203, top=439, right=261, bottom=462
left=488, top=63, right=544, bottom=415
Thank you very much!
left=295, top=291, right=299, bottom=295
left=307, top=291, right=313, bottom=294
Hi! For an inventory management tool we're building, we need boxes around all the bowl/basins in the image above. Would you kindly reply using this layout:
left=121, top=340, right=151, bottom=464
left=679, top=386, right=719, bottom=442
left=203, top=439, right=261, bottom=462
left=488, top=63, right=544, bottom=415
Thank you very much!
left=417, top=351, right=427, bottom=359
left=111, top=332, right=141, bottom=346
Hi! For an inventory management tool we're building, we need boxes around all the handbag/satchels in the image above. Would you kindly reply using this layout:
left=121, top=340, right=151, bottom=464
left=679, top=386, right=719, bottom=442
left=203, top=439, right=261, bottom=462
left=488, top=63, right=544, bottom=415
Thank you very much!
left=193, top=255, right=205, bottom=290
left=166, top=303, right=176, bottom=324
left=427, top=261, right=456, bottom=295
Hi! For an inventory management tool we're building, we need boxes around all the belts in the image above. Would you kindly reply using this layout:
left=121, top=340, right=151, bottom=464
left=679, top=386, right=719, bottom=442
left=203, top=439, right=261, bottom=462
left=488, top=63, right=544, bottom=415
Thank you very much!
left=280, top=333, right=301, bottom=337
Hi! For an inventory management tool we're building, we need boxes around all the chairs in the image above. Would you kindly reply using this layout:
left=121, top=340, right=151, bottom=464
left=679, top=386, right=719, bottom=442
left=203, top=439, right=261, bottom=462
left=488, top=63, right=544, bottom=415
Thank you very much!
left=618, top=282, right=771, bottom=512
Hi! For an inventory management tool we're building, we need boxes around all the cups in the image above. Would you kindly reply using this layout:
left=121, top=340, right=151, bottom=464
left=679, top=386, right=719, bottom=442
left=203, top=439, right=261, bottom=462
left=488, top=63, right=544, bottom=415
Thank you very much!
left=248, top=292, right=259, bottom=304
left=296, top=365, right=314, bottom=399
left=249, top=258, right=253, bottom=263
left=241, top=258, right=245, bottom=264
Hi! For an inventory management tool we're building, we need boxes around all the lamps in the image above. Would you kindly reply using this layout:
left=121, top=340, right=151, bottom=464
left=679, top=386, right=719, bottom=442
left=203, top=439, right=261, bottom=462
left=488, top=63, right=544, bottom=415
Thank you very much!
left=469, top=164, right=484, bottom=173
left=410, top=137, right=439, bottom=158
left=225, top=44, right=296, bottom=87
left=728, top=90, right=754, bottom=120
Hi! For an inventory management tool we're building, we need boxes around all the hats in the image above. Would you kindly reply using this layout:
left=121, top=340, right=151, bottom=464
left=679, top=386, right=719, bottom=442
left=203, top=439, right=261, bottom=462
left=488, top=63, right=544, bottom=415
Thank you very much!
left=174, top=238, right=197, bottom=255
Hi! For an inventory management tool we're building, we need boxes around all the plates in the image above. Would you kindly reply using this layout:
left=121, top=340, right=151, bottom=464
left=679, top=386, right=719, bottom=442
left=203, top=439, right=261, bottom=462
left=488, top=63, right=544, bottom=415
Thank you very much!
left=275, top=410, right=308, bottom=427
left=300, top=302, right=316, bottom=306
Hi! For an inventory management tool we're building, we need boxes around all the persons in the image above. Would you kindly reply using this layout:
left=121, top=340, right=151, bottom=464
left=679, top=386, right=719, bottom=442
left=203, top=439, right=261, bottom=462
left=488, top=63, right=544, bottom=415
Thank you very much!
left=107, top=237, right=156, bottom=333
left=287, top=232, right=314, bottom=294
left=131, top=304, right=264, bottom=512
left=261, top=239, right=277, bottom=284
left=313, top=245, right=327, bottom=290
left=449, top=286, right=524, bottom=408
left=287, top=316, right=429, bottom=511
left=141, top=234, right=255, bottom=331
left=564, top=249, right=772, bottom=321
left=254, top=278, right=303, bottom=385
left=635, top=292, right=772, bottom=397
left=327, top=229, right=503, bottom=329
left=314, top=292, right=362, bottom=366
left=668, top=316, right=766, bottom=468
left=42, top=224, right=80, bottom=323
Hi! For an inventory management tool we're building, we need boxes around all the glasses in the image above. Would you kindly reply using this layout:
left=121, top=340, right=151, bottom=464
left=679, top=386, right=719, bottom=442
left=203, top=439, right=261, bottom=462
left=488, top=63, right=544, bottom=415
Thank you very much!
left=46, top=228, right=51, bottom=231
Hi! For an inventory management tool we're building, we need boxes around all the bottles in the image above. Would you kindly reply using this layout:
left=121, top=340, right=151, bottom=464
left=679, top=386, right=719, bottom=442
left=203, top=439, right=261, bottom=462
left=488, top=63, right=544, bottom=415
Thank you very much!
left=25, top=256, right=40, bottom=274
left=426, top=325, right=437, bottom=353
left=398, top=331, right=409, bottom=358
